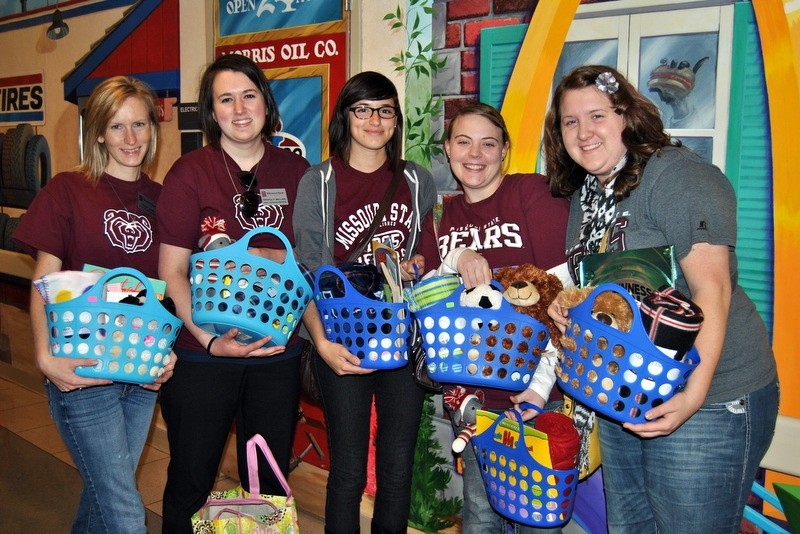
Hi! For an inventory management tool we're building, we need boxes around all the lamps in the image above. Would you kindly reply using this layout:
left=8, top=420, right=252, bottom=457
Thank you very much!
left=47, top=9, right=69, bottom=40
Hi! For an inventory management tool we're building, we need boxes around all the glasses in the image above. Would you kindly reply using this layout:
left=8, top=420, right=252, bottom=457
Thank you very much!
left=238, top=170, right=259, bottom=218
left=349, top=106, right=400, bottom=120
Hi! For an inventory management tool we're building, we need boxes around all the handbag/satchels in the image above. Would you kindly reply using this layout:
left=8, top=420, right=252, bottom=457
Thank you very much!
left=189, top=434, right=300, bottom=534
left=299, top=349, right=324, bottom=407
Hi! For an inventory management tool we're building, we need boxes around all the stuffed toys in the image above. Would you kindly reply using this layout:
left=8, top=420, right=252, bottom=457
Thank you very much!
left=554, top=285, right=634, bottom=414
left=195, top=214, right=231, bottom=282
left=443, top=384, right=487, bottom=454
left=461, top=262, right=563, bottom=378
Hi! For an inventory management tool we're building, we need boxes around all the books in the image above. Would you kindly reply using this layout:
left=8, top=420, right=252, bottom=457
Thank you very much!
left=31, top=264, right=167, bottom=305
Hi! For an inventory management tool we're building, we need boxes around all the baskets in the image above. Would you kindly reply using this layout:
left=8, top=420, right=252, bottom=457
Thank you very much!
left=556, top=282, right=701, bottom=427
left=415, top=279, right=550, bottom=392
left=470, top=403, right=580, bottom=529
left=189, top=227, right=314, bottom=348
left=45, top=267, right=183, bottom=385
left=315, top=265, right=410, bottom=369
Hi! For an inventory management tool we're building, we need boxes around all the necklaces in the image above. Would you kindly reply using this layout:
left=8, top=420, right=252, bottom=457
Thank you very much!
left=221, top=145, right=263, bottom=198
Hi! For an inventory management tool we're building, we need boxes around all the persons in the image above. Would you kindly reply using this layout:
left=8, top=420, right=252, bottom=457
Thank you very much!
left=542, top=65, right=782, bottom=534
left=431, top=101, right=570, bottom=534
left=10, top=75, right=183, bottom=534
left=290, top=71, right=438, bottom=533
left=103, top=169, right=140, bottom=227
left=151, top=53, right=314, bottom=534
